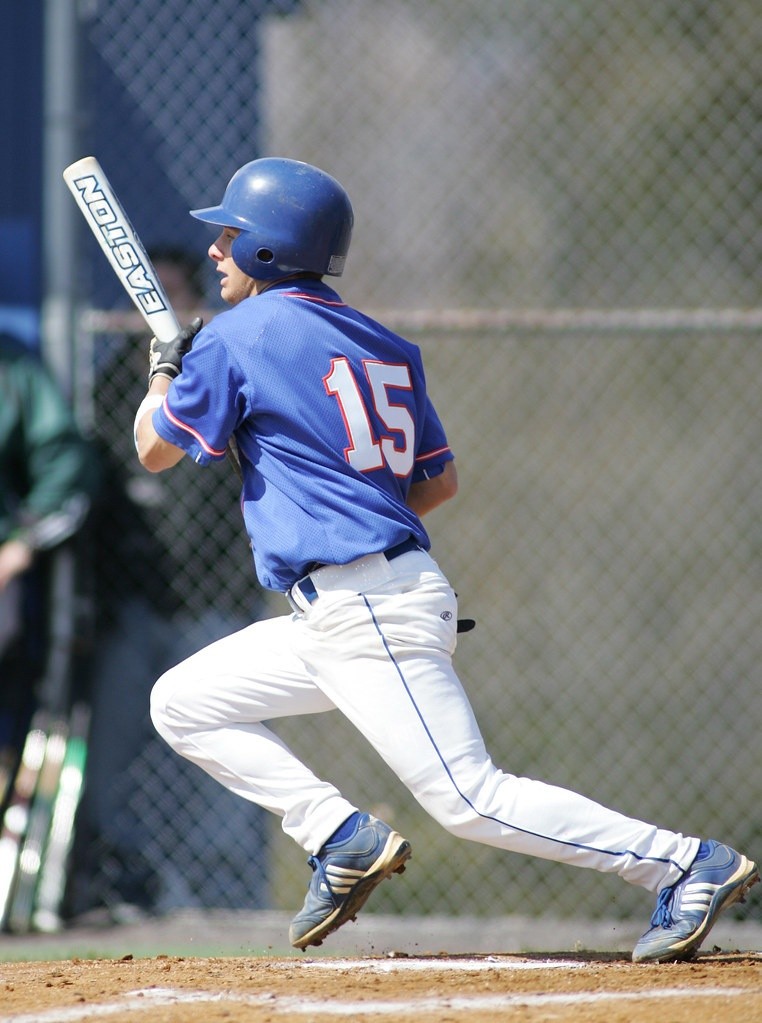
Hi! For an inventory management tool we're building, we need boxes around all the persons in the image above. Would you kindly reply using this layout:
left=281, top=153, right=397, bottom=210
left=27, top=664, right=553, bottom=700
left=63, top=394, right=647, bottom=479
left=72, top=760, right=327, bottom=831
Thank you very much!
left=0, top=225, right=267, bottom=929
left=132, top=157, right=760, bottom=964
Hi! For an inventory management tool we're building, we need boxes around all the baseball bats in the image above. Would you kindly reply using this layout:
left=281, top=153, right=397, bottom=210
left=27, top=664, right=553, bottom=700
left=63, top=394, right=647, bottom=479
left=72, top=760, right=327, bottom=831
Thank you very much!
left=60, top=152, right=183, bottom=344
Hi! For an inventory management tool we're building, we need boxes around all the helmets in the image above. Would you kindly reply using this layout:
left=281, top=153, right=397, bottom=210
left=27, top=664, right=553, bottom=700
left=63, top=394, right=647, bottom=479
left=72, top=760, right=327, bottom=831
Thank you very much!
left=189, top=157, right=355, bottom=282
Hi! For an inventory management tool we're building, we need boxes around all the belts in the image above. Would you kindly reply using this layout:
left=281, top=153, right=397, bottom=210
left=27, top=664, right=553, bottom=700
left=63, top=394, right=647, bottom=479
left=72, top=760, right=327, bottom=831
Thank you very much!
left=287, top=536, right=418, bottom=613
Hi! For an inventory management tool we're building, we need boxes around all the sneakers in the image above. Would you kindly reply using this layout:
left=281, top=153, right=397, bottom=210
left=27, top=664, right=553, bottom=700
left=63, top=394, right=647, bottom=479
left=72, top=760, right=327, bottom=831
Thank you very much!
left=290, top=811, right=412, bottom=952
left=630, top=839, right=762, bottom=963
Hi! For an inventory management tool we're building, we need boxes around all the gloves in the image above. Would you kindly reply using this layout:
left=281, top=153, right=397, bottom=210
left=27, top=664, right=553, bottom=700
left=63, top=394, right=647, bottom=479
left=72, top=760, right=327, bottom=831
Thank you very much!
left=145, top=316, right=204, bottom=385
